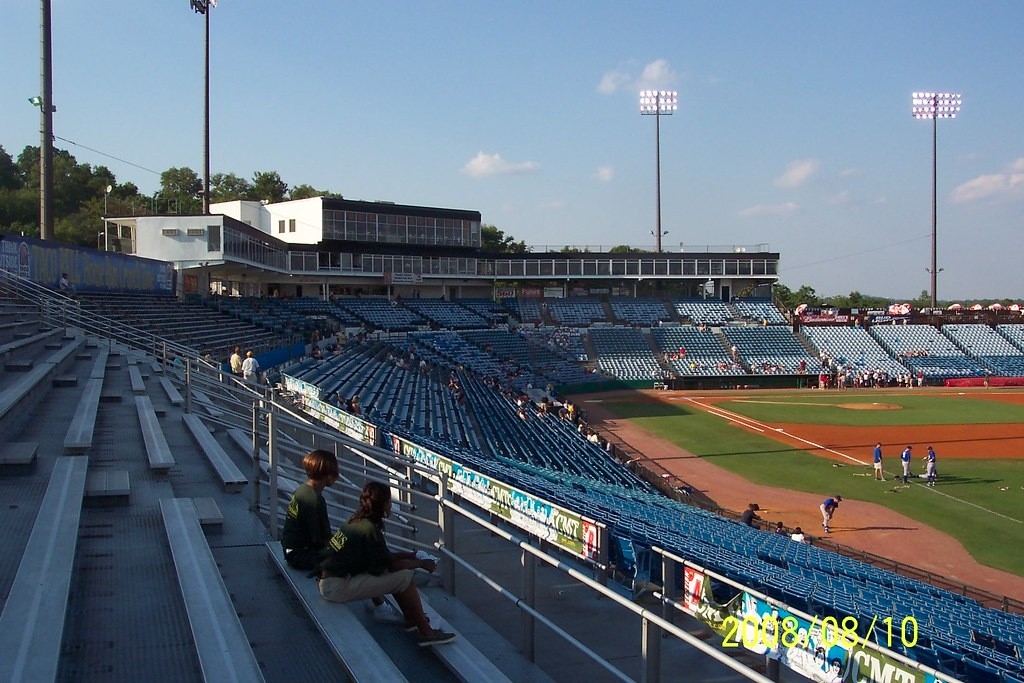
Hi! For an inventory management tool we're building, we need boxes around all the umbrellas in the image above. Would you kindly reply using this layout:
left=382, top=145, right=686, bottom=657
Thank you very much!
left=947, top=303, right=1022, bottom=311
left=795, top=303, right=807, bottom=316
left=890, top=303, right=911, bottom=314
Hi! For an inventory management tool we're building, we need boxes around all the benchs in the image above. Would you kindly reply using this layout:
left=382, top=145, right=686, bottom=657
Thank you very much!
left=0, top=293, right=480, bottom=683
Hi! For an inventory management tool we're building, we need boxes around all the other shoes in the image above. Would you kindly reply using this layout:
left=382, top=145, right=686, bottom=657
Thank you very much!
left=932, top=482, right=935, bottom=487
left=926, top=482, right=930, bottom=486
left=822, top=523, right=824, bottom=526
left=875, top=478, right=880, bottom=481
left=824, top=528, right=829, bottom=532
left=881, top=478, right=886, bottom=481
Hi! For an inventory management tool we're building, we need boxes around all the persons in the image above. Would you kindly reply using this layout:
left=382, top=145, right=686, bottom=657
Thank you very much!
left=819, top=495, right=843, bottom=534
left=775, top=521, right=806, bottom=544
left=59, top=273, right=77, bottom=295
left=900, top=446, right=913, bottom=483
left=174, top=346, right=261, bottom=390
left=280, top=450, right=457, bottom=647
left=823, top=308, right=835, bottom=316
left=383, top=289, right=531, bottom=419
left=537, top=396, right=670, bottom=482
left=338, top=395, right=364, bottom=418
left=984, top=373, right=989, bottom=390
left=923, top=446, right=936, bottom=486
left=213, top=292, right=366, bottom=359
left=527, top=317, right=930, bottom=390
left=873, top=442, right=888, bottom=481
left=740, top=503, right=761, bottom=530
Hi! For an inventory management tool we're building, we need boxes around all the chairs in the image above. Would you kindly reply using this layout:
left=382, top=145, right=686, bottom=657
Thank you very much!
left=215, top=300, right=1024, bottom=683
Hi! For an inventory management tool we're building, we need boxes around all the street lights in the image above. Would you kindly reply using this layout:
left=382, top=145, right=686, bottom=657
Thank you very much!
left=912, top=92, right=961, bottom=308
left=639, top=90, right=677, bottom=251
left=103, top=185, right=113, bottom=251
left=28, top=95, right=58, bottom=240
left=190, top=0, right=217, bottom=216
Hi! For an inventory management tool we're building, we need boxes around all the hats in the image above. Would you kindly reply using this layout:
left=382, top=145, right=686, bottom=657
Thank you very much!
left=836, top=495, right=842, bottom=501
left=246, top=351, right=253, bottom=356
left=926, top=446, right=932, bottom=450
left=907, top=446, right=912, bottom=449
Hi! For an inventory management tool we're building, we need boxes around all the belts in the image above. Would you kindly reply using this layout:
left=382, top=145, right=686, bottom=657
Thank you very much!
left=317, top=569, right=345, bottom=579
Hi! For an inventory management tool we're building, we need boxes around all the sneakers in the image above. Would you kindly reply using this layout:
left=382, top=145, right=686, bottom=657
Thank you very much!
left=417, top=628, right=455, bottom=647
left=403, top=612, right=430, bottom=632
